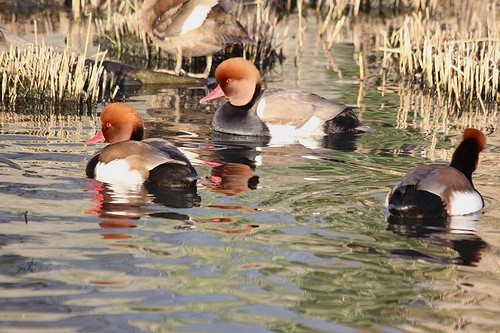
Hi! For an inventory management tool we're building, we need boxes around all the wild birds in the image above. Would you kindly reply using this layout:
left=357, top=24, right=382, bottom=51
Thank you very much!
left=137, top=0, right=257, bottom=79
left=385, top=128, right=487, bottom=224
left=197, top=57, right=374, bottom=137
left=83, top=103, right=197, bottom=189
